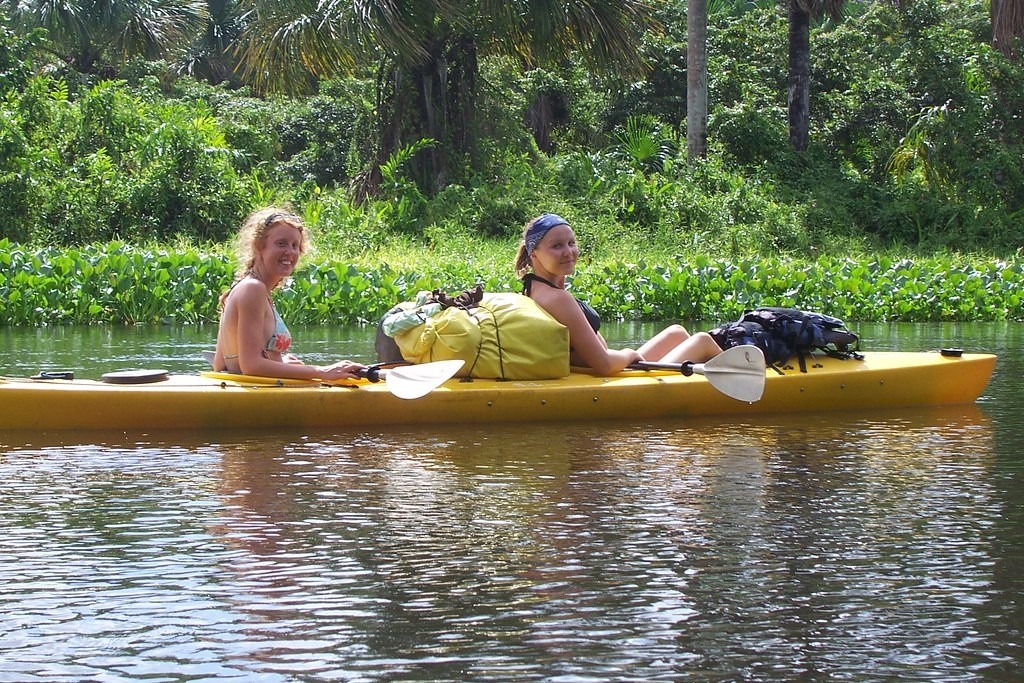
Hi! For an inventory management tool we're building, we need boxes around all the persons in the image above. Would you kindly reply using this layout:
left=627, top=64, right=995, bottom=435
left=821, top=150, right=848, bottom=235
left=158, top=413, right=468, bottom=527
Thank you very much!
left=214, top=210, right=366, bottom=380
left=516, top=211, right=724, bottom=375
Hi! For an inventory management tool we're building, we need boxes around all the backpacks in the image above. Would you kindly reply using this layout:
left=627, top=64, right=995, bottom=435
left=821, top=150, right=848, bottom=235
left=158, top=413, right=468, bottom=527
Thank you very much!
left=707, top=307, right=860, bottom=365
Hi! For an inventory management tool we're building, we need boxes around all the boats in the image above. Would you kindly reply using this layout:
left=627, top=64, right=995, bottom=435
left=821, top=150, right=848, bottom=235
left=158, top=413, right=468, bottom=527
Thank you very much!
left=0, top=347, right=997, bottom=426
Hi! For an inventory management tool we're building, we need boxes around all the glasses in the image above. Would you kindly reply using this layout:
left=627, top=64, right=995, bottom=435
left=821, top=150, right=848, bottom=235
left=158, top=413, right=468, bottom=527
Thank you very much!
left=264, top=213, right=300, bottom=226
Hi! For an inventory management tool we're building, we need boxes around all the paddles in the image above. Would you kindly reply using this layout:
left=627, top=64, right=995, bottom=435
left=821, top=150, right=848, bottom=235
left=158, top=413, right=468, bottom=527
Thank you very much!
left=626, top=344, right=766, bottom=402
left=352, top=359, right=466, bottom=400
